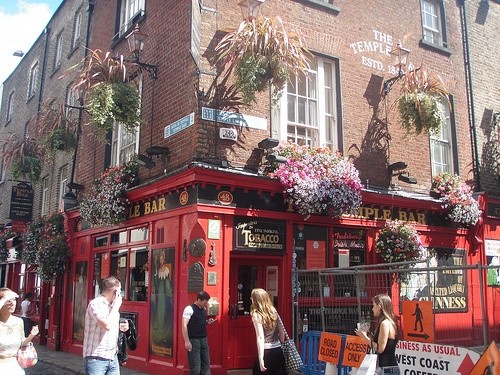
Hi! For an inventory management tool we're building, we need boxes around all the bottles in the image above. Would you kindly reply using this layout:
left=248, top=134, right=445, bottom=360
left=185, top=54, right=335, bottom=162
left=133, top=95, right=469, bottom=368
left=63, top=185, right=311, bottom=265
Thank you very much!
left=303, top=314, right=308, bottom=332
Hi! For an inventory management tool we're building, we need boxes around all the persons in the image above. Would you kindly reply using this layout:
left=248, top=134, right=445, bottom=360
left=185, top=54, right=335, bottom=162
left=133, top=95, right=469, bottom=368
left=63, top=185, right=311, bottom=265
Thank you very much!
left=355, top=294, right=400, bottom=375
left=0, top=287, right=39, bottom=375
left=182, top=291, right=217, bottom=375
left=20, top=292, right=33, bottom=337
left=250, top=288, right=288, bottom=375
left=82, top=276, right=129, bottom=375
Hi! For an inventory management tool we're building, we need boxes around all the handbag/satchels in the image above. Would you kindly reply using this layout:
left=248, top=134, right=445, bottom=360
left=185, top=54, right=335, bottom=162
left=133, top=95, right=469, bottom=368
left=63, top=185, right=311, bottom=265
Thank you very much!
left=26, top=304, right=37, bottom=317
left=281, top=339, right=304, bottom=373
left=355, top=341, right=378, bottom=375
left=17, top=341, right=38, bottom=369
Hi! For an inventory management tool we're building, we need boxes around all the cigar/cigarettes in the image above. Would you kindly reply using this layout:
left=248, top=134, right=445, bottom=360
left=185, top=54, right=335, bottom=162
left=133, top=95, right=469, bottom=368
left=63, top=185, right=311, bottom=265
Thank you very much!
left=189, top=350, right=190, bottom=351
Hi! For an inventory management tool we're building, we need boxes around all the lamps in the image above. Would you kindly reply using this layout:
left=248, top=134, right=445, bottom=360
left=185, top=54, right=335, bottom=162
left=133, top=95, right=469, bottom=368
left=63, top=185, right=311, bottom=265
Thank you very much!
left=255, top=138, right=288, bottom=164
left=124, top=22, right=150, bottom=62
left=387, top=161, right=418, bottom=184
left=237, top=0, right=265, bottom=25
left=133, top=146, right=169, bottom=167
left=384, top=38, right=423, bottom=98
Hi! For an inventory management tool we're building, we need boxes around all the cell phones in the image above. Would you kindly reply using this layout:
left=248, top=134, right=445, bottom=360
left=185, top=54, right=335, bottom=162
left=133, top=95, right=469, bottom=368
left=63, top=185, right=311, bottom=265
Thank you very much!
left=211, top=318, right=217, bottom=322
left=114, top=290, right=119, bottom=297
